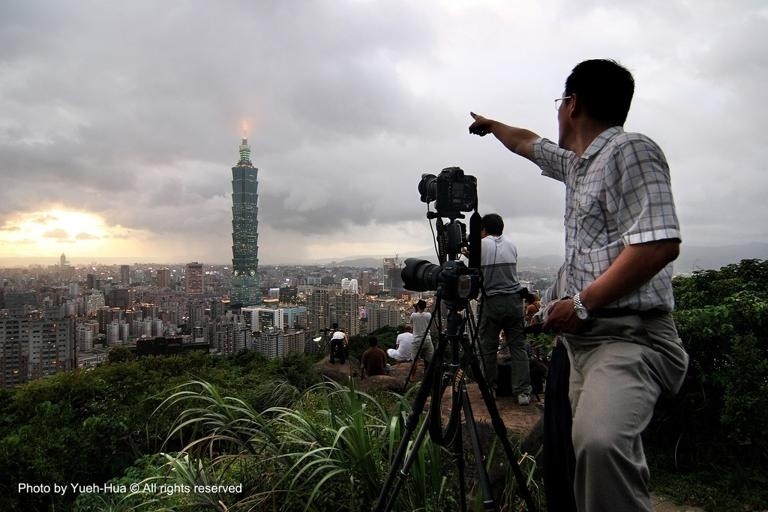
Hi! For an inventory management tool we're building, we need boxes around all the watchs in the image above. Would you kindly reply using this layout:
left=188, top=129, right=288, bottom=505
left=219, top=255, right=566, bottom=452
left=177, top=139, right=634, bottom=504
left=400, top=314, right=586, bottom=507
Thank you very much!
left=573, top=292, right=589, bottom=321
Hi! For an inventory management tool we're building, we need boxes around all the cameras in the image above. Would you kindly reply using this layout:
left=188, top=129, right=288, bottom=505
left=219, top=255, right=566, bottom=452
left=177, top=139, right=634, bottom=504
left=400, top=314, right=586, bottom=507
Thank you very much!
left=402, top=258, right=484, bottom=302
left=418, top=166, right=478, bottom=219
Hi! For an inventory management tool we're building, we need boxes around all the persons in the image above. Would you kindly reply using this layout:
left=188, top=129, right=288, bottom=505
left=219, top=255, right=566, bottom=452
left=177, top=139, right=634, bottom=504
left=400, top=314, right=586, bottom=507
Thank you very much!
left=467, top=59, right=693, bottom=512
left=329, top=293, right=540, bottom=379
left=475, top=213, right=534, bottom=406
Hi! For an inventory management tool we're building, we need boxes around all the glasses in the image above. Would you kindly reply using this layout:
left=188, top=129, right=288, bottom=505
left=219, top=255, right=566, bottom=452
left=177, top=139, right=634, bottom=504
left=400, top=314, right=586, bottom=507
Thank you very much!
left=555, top=96, right=571, bottom=110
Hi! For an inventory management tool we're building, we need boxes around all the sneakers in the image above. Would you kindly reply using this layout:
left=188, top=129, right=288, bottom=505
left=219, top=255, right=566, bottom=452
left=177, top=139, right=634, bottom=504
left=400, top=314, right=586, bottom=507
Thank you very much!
left=518, top=393, right=531, bottom=405
left=489, top=388, right=496, bottom=399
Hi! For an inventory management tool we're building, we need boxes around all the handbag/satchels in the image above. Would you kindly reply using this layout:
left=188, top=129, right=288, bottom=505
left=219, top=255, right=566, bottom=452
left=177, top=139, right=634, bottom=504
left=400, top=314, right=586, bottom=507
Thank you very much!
left=498, top=356, right=543, bottom=396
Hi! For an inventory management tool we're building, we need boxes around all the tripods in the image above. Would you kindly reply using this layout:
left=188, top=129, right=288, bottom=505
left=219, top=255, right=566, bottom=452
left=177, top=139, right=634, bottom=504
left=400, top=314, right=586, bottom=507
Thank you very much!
left=375, top=331, right=537, bottom=511
left=402, top=293, right=496, bottom=400
left=318, top=336, right=348, bottom=353
